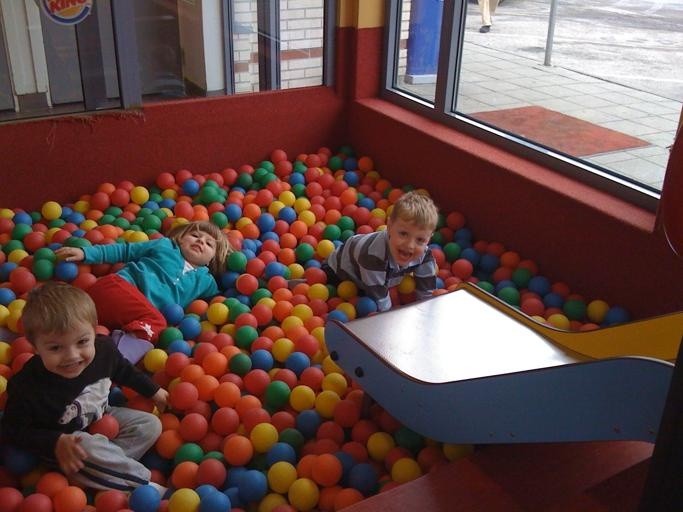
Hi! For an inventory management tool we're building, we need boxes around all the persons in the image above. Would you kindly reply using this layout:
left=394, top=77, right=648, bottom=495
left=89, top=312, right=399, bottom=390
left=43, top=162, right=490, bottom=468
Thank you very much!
left=477, top=0, right=499, bottom=34
left=319, top=190, right=439, bottom=315
left=54, top=219, right=230, bottom=405
left=1, top=282, right=174, bottom=500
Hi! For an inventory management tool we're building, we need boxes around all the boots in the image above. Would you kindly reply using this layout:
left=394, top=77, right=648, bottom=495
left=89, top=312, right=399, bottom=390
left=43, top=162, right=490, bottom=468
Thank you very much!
left=108, top=330, right=155, bottom=405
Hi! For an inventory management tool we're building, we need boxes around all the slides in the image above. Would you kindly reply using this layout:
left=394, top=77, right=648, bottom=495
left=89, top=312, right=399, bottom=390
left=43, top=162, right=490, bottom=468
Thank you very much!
left=324, top=280, right=683, bottom=444
